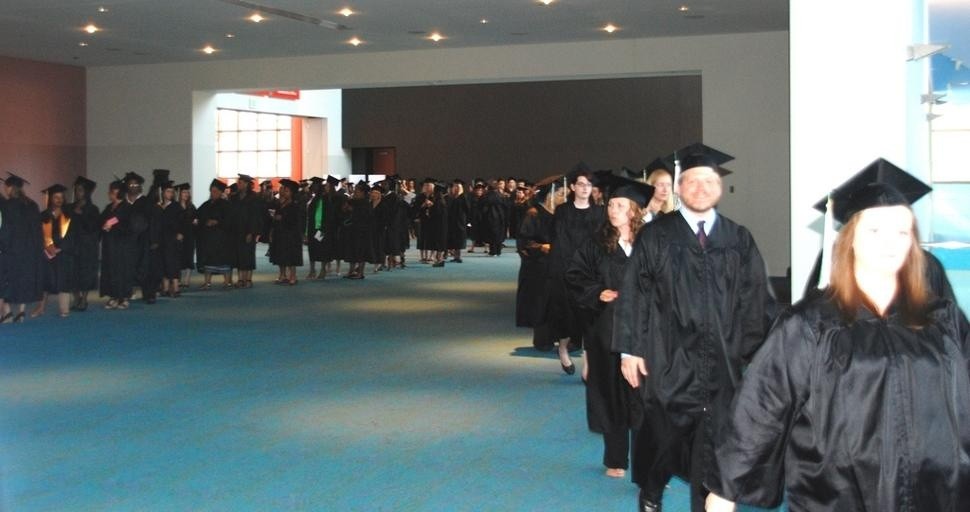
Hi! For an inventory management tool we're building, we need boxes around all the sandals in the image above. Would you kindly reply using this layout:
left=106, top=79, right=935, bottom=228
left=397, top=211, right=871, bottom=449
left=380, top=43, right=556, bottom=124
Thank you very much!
left=272, top=258, right=407, bottom=285
left=71, top=281, right=189, bottom=311
left=199, top=280, right=252, bottom=290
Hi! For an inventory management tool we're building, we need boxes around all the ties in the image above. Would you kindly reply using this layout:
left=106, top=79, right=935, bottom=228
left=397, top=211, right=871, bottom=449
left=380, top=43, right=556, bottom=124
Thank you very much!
left=697, top=221, right=707, bottom=248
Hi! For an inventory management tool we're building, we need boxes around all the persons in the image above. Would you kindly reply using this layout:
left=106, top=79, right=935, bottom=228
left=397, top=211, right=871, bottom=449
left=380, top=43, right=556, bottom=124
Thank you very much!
left=807, top=217, right=968, bottom=342
left=611, top=145, right=777, bottom=511
left=1, top=162, right=535, bottom=326
left=514, top=162, right=674, bottom=477
left=702, top=158, right=970, bottom=510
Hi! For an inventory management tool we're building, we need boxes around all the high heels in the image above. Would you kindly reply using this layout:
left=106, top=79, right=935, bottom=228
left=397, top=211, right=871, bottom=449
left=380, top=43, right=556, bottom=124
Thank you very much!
left=0, top=312, right=13, bottom=323
left=15, top=312, right=25, bottom=322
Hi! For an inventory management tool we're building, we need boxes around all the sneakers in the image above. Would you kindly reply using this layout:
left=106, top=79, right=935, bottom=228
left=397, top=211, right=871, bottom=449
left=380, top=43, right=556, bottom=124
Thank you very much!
left=639, top=493, right=662, bottom=512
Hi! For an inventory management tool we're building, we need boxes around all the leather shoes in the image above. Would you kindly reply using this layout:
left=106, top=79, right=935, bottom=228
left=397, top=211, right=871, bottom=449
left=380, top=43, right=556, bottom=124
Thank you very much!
left=557, top=345, right=576, bottom=375
left=59, top=312, right=69, bottom=317
left=30, top=308, right=44, bottom=318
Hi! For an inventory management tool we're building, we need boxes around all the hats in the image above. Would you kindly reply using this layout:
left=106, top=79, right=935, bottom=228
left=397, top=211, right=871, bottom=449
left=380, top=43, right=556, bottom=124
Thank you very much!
left=157, top=180, right=176, bottom=205
left=40, top=183, right=65, bottom=194
left=5, top=170, right=30, bottom=188
left=424, top=177, right=487, bottom=190
left=210, top=179, right=227, bottom=193
left=592, top=142, right=735, bottom=211
left=516, top=186, right=528, bottom=193
left=534, top=173, right=571, bottom=212
left=226, top=174, right=399, bottom=197
left=74, top=175, right=96, bottom=191
left=174, top=182, right=191, bottom=203
left=814, top=156, right=935, bottom=293
